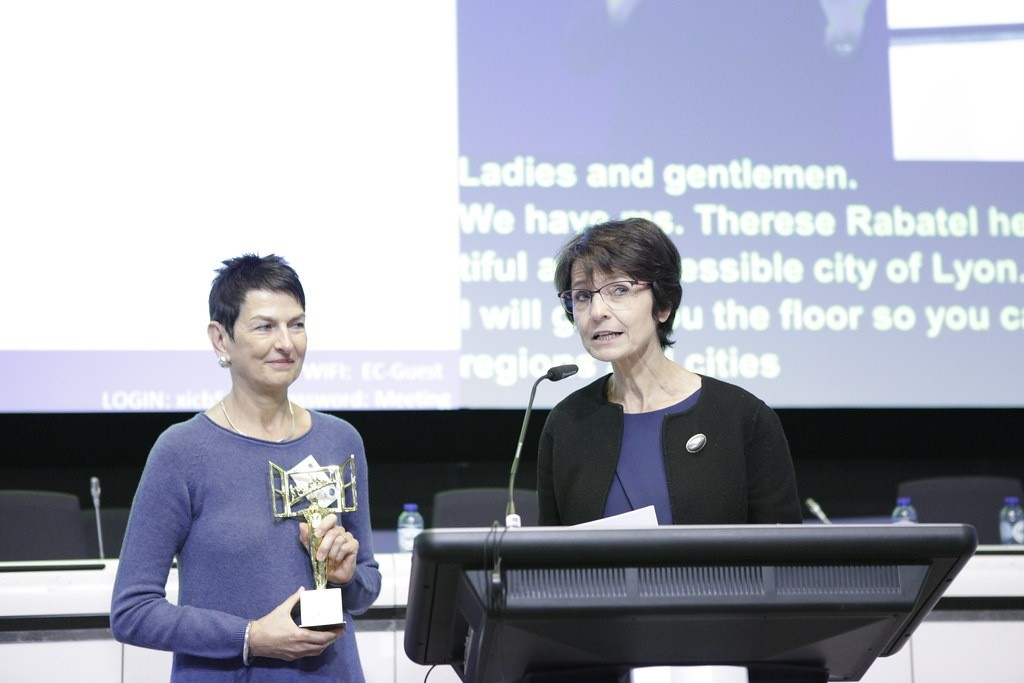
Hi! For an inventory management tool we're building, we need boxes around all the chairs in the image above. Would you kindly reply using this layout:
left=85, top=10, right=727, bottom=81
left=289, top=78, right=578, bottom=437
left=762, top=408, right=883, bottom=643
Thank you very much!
left=433, top=488, right=538, bottom=526
left=897, top=474, right=1023, bottom=544
left=0, top=476, right=104, bottom=562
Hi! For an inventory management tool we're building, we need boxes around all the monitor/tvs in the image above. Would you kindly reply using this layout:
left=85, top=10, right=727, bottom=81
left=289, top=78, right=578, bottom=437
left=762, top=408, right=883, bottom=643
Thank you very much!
left=405, top=518, right=981, bottom=683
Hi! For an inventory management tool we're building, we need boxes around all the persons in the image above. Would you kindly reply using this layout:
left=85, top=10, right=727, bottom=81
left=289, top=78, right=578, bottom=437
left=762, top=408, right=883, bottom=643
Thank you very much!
left=535, top=214, right=803, bottom=526
left=109, top=249, right=384, bottom=683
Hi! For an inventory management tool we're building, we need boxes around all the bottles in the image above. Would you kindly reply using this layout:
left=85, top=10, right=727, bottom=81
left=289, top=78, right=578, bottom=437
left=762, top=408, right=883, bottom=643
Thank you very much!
left=999, top=498, right=1024, bottom=543
left=398, top=504, right=423, bottom=552
left=892, top=497, right=918, bottom=525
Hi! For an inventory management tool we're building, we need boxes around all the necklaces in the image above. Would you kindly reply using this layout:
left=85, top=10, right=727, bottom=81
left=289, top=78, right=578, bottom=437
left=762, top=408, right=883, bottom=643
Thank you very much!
left=219, top=395, right=299, bottom=443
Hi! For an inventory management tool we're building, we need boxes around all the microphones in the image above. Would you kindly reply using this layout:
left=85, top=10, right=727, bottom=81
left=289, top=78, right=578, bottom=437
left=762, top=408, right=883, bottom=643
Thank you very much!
left=91, top=476, right=105, bottom=559
left=505, top=364, right=579, bottom=528
left=805, top=496, right=832, bottom=525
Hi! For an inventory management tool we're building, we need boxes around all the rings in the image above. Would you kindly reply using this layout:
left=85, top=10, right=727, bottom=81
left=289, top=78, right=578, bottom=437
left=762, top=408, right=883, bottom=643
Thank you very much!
left=341, top=533, right=347, bottom=542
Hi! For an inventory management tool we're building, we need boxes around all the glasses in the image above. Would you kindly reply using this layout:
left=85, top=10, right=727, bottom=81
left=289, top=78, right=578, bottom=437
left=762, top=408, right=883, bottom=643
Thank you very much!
left=558, top=280, right=654, bottom=314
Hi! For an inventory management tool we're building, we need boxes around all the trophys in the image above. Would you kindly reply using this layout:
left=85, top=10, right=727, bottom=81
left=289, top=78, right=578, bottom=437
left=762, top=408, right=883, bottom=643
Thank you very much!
left=266, top=452, right=361, bottom=629
